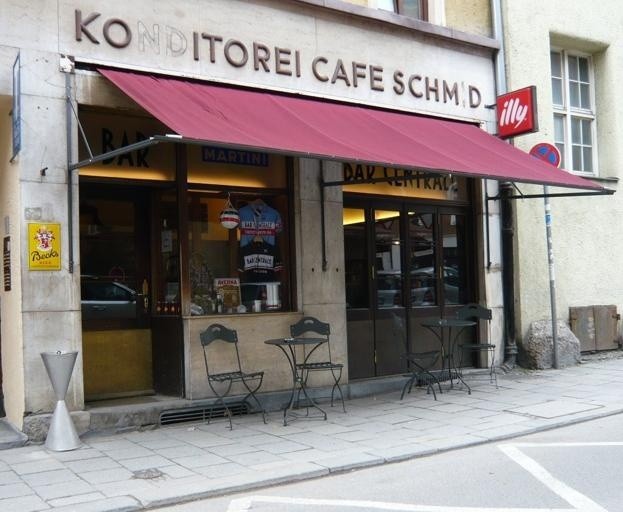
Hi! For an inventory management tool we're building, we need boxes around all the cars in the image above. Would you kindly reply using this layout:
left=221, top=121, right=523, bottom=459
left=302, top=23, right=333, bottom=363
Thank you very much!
left=82, top=275, right=203, bottom=315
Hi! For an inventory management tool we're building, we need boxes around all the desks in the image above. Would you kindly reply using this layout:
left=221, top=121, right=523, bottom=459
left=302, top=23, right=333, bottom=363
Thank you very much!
left=263, top=337, right=329, bottom=426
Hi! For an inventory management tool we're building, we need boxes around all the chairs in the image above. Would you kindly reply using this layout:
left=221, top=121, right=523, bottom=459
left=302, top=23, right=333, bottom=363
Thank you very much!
left=289, top=316, right=348, bottom=417
left=390, top=303, right=499, bottom=404
left=198, top=324, right=268, bottom=430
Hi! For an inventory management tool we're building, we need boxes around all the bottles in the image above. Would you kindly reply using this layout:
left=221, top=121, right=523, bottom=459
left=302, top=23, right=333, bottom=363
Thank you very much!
left=215, top=293, right=224, bottom=314
left=156, top=300, right=178, bottom=315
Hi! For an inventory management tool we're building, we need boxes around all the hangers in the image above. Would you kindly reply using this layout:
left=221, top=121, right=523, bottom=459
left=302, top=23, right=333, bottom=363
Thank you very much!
left=252, top=232, right=264, bottom=243
left=252, top=195, right=267, bottom=206
